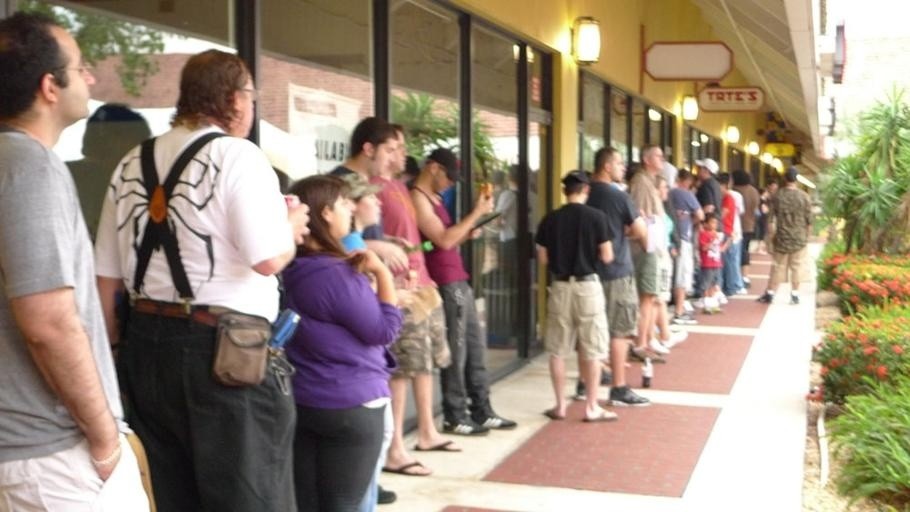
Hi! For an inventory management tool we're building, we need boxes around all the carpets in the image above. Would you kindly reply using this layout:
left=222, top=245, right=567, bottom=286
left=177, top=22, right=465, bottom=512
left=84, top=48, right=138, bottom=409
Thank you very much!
left=668, top=251, right=781, bottom=328
left=439, top=503, right=522, bottom=512
left=610, top=332, right=754, bottom=396
left=480, top=398, right=723, bottom=499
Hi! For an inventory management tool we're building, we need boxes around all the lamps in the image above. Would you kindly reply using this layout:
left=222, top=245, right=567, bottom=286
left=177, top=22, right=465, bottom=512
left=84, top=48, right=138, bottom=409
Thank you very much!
left=680, top=93, right=700, bottom=123
left=569, top=15, right=604, bottom=67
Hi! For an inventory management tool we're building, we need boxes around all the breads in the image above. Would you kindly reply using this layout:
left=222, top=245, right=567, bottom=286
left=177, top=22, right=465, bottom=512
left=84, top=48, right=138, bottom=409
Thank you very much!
left=479, top=183, right=492, bottom=200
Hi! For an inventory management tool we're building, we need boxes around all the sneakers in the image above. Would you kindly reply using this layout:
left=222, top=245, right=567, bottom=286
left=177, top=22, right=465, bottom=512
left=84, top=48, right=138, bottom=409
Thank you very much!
left=700, top=278, right=751, bottom=314
left=377, top=484, right=396, bottom=504
left=442, top=413, right=490, bottom=436
left=790, top=295, right=800, bottom=305
left=758, top=291, right=774, bottom=303
left=674, top=312, right=697, bottom=325
left=610, top=385, right=649, bottom=405
left=575, top=381, right=591, bottom=401
left=470, top=411, right=517, bottom=430
left=627, top=331, right=688, bottom=364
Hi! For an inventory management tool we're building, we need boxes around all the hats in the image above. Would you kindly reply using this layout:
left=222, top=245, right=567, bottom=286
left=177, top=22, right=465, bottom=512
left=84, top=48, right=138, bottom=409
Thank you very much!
left=561, top=169, right=591, bottom=186
left=338, top=171, right=382, bottom=200
left=694, top=158, right=719, bottom=175
left=428, top=148, right=463, bottom=182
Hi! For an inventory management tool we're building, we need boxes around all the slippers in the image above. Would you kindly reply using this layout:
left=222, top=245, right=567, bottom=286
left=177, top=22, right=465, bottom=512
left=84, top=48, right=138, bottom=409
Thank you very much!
left=381, top=460, right=431, bottom=477
left=546, top=410, right=563, bottom=420
left=582, top=409, right=619, bottom=423
left=415, top=440, right=462, bottom=452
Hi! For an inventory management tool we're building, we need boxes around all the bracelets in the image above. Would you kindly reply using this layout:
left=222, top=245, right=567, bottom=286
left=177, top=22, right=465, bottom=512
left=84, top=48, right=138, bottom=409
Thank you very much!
left=93, top=446, right=121, bottom=465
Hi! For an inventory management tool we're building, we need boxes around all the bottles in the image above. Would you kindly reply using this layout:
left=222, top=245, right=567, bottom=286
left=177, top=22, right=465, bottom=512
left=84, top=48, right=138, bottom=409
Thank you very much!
left=267, top=305, right=302, bottom=350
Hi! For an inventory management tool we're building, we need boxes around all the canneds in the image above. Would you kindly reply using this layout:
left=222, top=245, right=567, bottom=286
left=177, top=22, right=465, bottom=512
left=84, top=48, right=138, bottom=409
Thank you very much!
left=285, top=194, right=301, bottom=210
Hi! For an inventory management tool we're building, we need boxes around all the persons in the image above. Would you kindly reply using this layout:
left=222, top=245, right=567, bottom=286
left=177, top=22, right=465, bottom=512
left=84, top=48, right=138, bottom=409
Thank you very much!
left=93, top=49, right=302, bottom=511
left=0, top=8, right=150, bottom=511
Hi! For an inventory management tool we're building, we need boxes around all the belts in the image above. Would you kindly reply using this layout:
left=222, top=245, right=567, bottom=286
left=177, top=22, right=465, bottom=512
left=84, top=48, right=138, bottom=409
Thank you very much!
left=554, top=274, right=597, bottom=282
left=133, top=298, right=218, bottom=329
